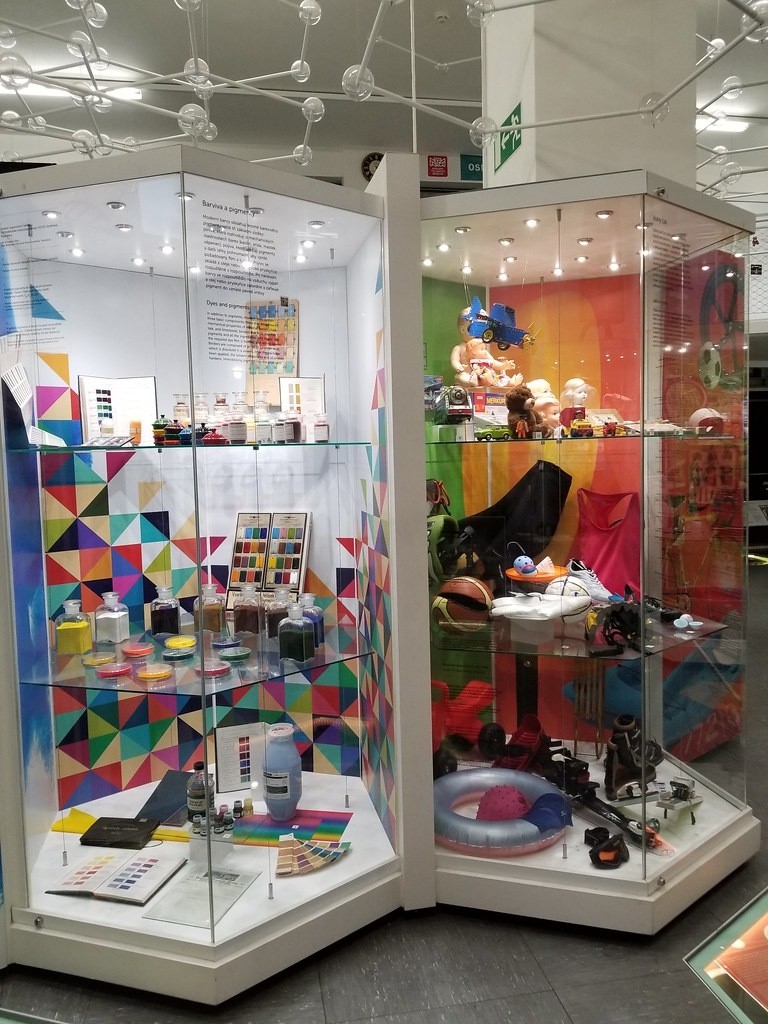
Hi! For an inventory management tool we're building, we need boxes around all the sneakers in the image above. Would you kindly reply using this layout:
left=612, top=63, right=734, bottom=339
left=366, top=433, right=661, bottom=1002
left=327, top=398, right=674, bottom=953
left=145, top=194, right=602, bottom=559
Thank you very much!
left=532, top=736, right=590, bottom=778
left=640, top=595, right=682, bottom=620
left=623, top=583, right=640, bottom=607
left=565, top=557, right=614, bottom=603
left=491, top=591, right=592, bottom=624
left=584, top=607, right=627, bottom=657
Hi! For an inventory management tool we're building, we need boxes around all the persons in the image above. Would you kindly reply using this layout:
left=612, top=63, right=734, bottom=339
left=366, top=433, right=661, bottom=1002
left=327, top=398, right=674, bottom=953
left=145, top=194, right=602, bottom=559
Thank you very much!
left=451, top=305, right=523, bottom=386
left=527, top=379, right=588, bottom=428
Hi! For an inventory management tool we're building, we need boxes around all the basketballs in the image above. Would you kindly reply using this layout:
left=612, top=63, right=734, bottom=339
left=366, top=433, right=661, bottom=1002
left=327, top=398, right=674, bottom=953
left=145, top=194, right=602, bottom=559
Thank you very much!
left=688, top=407, right=724, bottom=434
left=430, top=574, right=497, bottom=637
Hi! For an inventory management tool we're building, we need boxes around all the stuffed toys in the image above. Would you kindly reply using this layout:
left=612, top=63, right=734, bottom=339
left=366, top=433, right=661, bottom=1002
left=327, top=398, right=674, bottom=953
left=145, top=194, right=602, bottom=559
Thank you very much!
left=504, top=385, right=554, bottom=438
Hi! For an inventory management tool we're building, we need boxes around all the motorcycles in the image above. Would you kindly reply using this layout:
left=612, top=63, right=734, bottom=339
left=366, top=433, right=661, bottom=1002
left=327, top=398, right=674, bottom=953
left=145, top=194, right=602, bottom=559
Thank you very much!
left=601, top=419, right=618, bottom=436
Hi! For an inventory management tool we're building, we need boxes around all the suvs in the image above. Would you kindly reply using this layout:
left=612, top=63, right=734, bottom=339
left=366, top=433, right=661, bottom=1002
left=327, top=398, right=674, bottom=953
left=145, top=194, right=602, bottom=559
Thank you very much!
left=474, top=424, right=511, bottom=441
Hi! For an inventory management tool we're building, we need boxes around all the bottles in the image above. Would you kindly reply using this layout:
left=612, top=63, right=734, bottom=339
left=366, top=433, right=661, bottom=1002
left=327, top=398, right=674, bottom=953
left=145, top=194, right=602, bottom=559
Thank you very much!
left=208, top=807, right=216, bottom=823
left=233, top=801, right=244, bottom=819
left=278, top=604, right=316, bottom=664
left=172, top=392, right=329, bottom=445
left=95, top=592, right=130, bottom=643
left=192, top=815, right=202, bottom=834
left=300, top=592, right=326, bottom=649
left=220, top=805, right=228, bottom=816
left=213, top=817, right=225, bottom=834
left=234, top=584, right=266, bottom=636
left=55, top=600, right=92, bottom=654
left=194, top=584, right=227, bottom=640
left=150, top=586, right=180, bottom=635
left=129, top=420, right=141, bottom=446
left=267, top=587, right=297, bottom=640
left=187, top=762, right=215, bottom=821
left=244, top=798, right=253, bottom=816
left=200, top=817, right=211, bottom=836
left=223, top=811, right=234, bottom=831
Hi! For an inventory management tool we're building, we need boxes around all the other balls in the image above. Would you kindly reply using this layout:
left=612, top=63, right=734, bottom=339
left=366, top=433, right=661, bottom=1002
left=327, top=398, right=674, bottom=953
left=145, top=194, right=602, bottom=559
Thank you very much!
left=520, top=565, right=538, bottom=575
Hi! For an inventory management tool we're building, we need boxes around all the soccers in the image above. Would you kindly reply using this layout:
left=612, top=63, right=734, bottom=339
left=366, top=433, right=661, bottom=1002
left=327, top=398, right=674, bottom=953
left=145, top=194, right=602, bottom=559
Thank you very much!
left=544, top=575, right=593, bottom=625
left=513, top=555, right=535, bottom=575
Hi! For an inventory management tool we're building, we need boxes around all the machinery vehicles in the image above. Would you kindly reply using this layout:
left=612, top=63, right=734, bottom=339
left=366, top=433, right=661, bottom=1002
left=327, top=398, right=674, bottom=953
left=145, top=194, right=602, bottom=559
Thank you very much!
left=559, top=408, right=593, bottom=438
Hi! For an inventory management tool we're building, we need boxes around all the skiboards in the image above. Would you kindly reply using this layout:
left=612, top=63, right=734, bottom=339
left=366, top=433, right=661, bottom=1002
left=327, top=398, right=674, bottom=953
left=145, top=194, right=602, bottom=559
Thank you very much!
left=490, top=715, right=679, bottom=861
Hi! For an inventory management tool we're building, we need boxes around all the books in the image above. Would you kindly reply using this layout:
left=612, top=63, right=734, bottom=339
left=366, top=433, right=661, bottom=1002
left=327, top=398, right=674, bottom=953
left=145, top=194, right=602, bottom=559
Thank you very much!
left=45, top=851, right=189, bottom=907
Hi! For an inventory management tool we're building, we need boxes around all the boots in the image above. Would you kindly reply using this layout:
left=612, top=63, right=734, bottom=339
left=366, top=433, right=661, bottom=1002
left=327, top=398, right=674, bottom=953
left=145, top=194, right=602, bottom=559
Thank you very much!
left=602, top=731, right=657, bottom=801
left=612, top=713, right=664, bottom=766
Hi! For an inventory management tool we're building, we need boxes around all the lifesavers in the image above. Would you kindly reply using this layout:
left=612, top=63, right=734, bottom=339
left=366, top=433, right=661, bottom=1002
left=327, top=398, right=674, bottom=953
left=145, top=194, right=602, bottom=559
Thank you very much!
left=432, top=766, right=573, bottom=858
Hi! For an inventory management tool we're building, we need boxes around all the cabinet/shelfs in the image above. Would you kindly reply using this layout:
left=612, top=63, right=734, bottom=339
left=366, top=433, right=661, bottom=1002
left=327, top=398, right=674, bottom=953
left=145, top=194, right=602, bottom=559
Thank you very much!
left=0, top=145, right=436, bottom=1007
left=420, top=169, right=757, bottom=937
